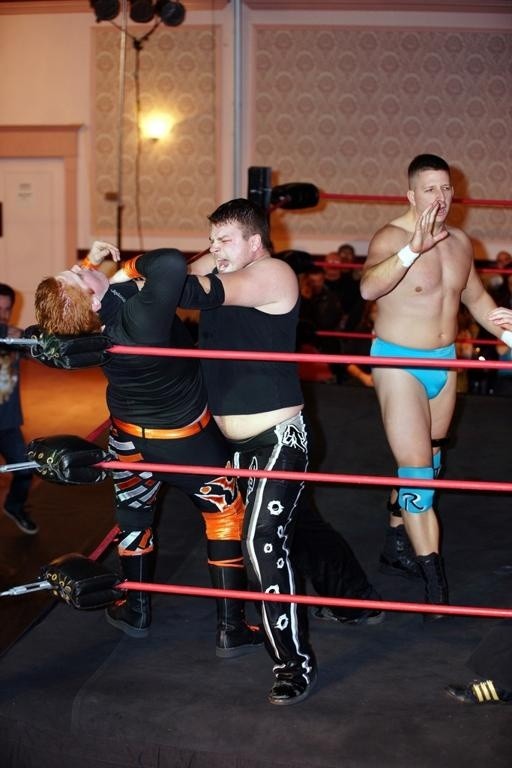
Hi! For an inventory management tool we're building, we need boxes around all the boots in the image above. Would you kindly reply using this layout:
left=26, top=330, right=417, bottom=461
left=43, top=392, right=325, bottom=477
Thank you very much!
left=209, top=564, right=265, bottom=657
left=105, top=550, right=151, bottom=638
left=418, top=552, right=449, bottom=623
left=379, top=525, right=424, bottom=580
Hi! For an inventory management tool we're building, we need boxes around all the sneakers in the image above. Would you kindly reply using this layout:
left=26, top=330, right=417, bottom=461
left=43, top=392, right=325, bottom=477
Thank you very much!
left=311, top=606, right=385, bottom=624
left=3, top=500, right=39, bottom=534
left=443, top=679, right=512, bottom=706
left=269, top=679, right=310, bottom=705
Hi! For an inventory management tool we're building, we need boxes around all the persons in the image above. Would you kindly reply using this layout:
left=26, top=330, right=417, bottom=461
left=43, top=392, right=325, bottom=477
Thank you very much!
left=273, top=241, right=512, bottom=388
left=0, top=282, right=41, bottom=535
left=35, top=239, right=266, bottom=656
left=360, top=153, right=512, bottom=622
left=97, top=198, right=320, bottom=704
